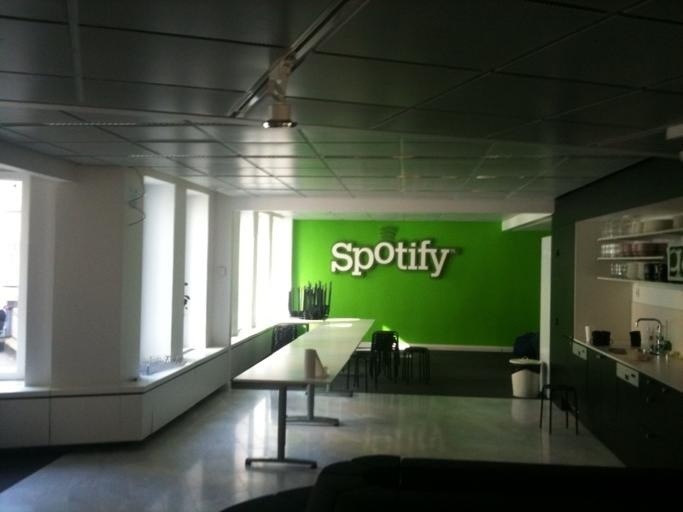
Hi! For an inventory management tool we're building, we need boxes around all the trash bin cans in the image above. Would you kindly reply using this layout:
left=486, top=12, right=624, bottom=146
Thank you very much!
left=509, top=355, right=542, bottom=399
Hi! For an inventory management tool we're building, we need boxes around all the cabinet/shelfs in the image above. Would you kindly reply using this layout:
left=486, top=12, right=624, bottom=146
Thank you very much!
left=552, top=335, right=683, bottom=469
left=595, top=226, right=683, bottom=287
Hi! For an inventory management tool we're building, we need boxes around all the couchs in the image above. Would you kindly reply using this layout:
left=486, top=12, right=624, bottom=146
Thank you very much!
left=218, top=456, right=682, bottom=512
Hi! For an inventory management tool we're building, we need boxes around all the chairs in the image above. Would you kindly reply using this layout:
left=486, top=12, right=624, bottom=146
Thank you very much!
left=347, top=330, right=430, bottom=396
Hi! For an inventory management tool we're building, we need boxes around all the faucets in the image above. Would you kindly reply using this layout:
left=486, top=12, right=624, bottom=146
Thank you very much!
left=635, top=319, right=664, bottom=351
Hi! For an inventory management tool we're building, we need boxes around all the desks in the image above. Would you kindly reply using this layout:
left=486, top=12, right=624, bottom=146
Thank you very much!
left=233, top=318, right=376, bottom=470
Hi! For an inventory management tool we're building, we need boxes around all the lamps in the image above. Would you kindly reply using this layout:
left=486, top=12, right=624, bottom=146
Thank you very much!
left=265, top=53, right=296, bottom=128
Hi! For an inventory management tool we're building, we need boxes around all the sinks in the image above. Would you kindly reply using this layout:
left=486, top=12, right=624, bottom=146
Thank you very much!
left=608, top=345, right=647, bottom=357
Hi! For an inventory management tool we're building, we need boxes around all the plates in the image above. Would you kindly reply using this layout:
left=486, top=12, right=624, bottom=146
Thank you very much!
left=632, top=214, right=683, bottom=233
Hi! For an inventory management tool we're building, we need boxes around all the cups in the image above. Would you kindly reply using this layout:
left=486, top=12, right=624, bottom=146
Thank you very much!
left=630, top=331, right=641, bottom=347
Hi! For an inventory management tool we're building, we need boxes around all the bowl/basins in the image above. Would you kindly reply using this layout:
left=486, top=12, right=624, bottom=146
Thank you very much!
left=626, top=261, right=646, bottom=281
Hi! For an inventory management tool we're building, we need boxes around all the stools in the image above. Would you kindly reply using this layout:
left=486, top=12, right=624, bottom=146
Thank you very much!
left=290, top=281, right=332, bottom=320
left=272, top=325, right=306, bottom=353
left=539, top=385, right=578, bottom=435
